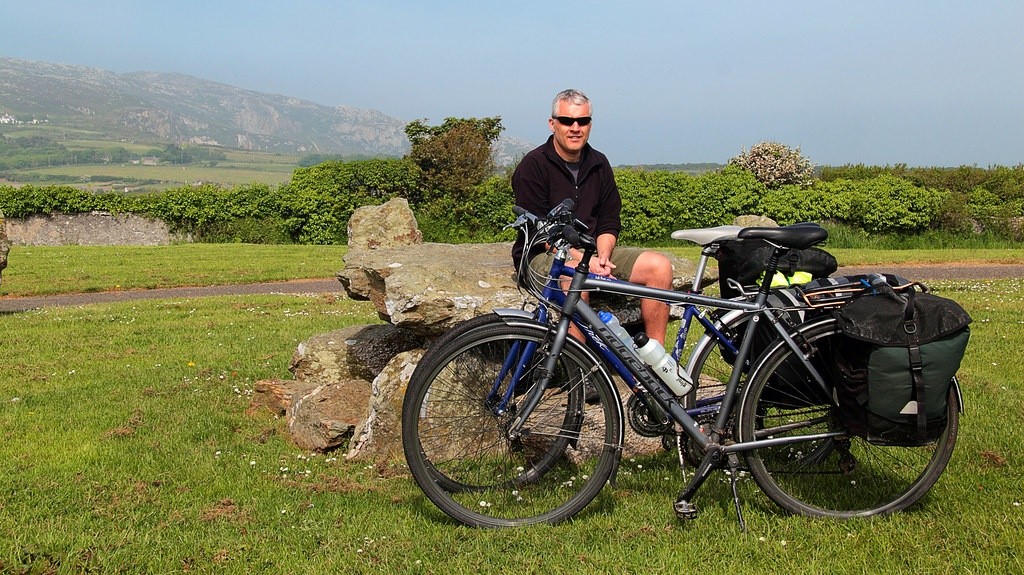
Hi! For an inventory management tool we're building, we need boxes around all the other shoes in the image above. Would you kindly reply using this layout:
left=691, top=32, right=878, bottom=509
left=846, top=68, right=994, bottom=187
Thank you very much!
left=585, top=378, right=598, bottom=402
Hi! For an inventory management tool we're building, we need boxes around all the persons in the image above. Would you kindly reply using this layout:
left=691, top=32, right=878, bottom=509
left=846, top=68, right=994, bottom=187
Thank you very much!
left=511, top=89, right=673, bottom=406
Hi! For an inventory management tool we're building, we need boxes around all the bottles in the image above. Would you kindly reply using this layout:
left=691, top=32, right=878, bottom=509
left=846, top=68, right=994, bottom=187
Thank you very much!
left=598, top=311, right=645, bottom=365
left=634, top=332, right=693, bottom=396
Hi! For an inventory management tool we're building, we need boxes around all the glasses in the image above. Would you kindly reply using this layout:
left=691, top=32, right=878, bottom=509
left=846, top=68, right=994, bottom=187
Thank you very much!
left=552, top=116, right=591, bottom=126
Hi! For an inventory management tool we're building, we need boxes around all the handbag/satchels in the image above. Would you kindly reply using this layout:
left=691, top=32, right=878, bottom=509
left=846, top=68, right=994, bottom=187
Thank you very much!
left=832, top=287, right=973, bottom=446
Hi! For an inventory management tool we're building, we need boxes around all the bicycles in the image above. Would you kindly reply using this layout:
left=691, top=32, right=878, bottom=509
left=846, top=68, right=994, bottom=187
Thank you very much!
left=403, top=199, right=965, bottom=529
left=404, top=205, right=840, bottom=495
